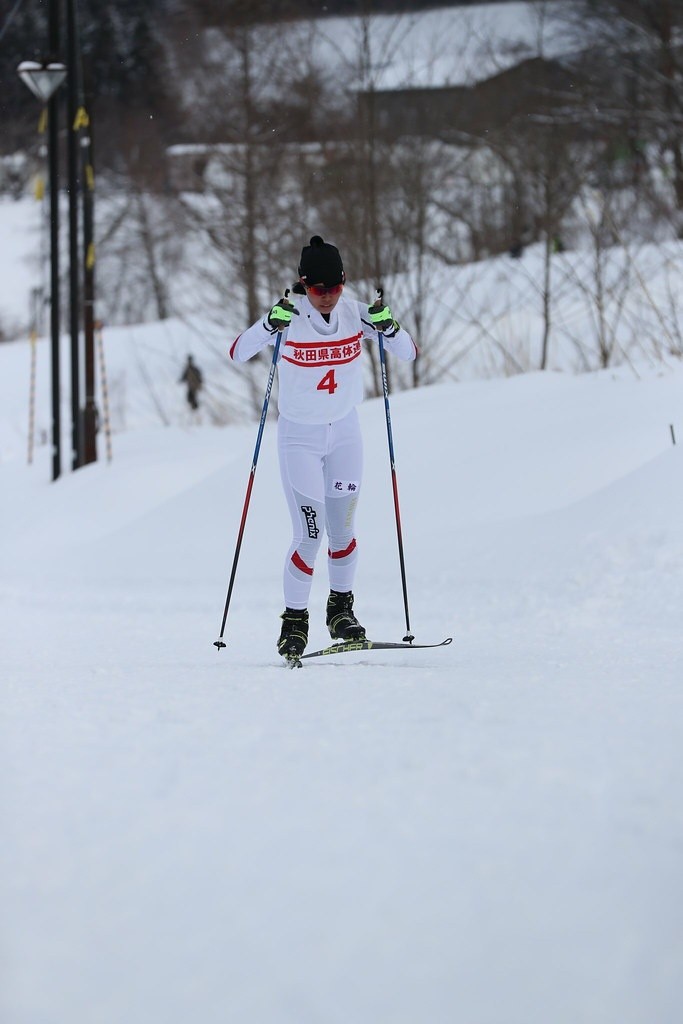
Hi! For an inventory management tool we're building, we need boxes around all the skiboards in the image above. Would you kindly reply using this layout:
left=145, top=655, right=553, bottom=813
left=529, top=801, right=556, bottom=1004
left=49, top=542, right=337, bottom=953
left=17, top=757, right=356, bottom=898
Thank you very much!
left=285, top=637, right=453, bottom=669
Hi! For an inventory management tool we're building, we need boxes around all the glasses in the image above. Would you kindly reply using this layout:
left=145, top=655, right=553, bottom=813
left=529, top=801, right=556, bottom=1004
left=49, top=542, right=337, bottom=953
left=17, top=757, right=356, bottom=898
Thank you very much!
left=308, top=283, right=342, bottom=296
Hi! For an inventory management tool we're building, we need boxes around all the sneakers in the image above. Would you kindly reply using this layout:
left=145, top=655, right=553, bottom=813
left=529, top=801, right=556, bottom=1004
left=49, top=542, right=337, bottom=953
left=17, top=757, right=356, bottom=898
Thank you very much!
left=325, top=594, right=365, bottom=641
left=276, top=610, right=309, bottom=659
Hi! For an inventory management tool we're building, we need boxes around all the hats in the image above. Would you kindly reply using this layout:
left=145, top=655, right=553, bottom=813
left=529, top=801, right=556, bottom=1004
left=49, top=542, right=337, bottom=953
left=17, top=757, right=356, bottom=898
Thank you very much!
left=298, top=236, right=346, bottom=286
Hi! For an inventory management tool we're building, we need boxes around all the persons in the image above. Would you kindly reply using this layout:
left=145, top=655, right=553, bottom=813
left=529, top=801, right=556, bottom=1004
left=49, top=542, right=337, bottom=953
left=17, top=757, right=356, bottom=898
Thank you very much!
left=178, top=352, right=208, bottom=409
left=228, top=243, right=420, bottom=657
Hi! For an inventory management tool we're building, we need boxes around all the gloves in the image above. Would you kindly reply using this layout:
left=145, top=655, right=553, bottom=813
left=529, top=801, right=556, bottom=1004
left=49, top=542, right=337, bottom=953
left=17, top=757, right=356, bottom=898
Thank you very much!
left=268, top=298, right=300, bottom=328
left=367, top=302, right=400, bottom=337
